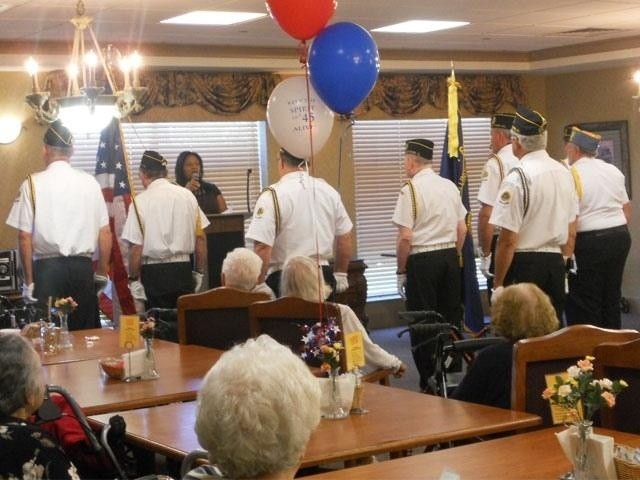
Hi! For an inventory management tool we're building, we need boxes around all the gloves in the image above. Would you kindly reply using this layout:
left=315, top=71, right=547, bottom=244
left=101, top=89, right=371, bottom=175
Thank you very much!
left=478, top=246, right=504, bottom=306
left=192, top=271, right=203, bottom=291
left=563, top=255, right=577, bottom=274
left=94, top=275, right=109, bottom=297
left=23, top=283, right=38, bottom=304
left=333, top=272, right=348, bottom=291
left=397, top=272, right=407, bottom=299
left=130, top=281, right=147, bottom=300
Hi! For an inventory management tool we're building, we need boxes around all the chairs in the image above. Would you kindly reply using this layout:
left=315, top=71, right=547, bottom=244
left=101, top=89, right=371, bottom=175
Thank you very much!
left=249, top=296, right=391, bottom=386
left=595, top=339, right=638, bottom=434
left=176, top=286, right=272, bottom=352
left=510, top=325, right=639, bottom=415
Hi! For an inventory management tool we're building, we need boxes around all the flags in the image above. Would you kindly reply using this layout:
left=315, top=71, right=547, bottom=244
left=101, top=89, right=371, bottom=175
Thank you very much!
left=439, top=79, right=484, bottom=337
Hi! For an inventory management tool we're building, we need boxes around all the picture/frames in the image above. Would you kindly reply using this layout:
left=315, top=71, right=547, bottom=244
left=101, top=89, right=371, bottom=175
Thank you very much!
left=572, top=117, right=634, bottom=201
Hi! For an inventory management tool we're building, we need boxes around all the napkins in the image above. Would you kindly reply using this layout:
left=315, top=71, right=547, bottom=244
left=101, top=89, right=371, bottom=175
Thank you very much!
left=314, top=373, right=356, bottom=416
left=554, top=425, right=615, bottom=476
left=122, top=348, right=146, bottom=381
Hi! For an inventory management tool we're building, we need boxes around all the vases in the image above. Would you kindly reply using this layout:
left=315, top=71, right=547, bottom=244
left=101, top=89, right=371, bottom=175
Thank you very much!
left=328, top=368, right=342, bottom=419
left=574, top=422, right=592, bottom=475
left=144, top=346, right=156, bottom=381
left=60, top=320, right=70, bottom=350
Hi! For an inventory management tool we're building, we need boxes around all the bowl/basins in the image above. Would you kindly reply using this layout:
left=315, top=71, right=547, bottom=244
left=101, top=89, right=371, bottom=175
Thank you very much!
left=99, top=354, right=125, bottom=380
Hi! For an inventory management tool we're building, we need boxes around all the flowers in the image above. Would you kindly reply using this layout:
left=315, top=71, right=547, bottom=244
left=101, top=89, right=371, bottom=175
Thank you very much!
left=300, top=315, right=342, bottom=368
left=542, top=356, right=629, bottom=430
left=55, top=295, right=78, bottom=320
left=140, top=316, right=156, bottom=345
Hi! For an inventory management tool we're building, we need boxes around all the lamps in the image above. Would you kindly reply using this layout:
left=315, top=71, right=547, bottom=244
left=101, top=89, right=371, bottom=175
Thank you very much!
left=19, top=1, right=153, bottom=138
left=0, top=114, right=20, bottom=146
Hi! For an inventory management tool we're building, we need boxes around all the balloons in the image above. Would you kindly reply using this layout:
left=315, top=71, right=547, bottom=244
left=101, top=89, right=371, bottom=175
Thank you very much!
left=264, top=1, right=381, bottom=162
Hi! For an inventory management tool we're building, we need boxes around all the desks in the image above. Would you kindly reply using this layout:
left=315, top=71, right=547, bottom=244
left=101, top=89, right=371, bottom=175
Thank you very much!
left=87, top=377, right=543, bottom=474
left=304, top=423, right=638, bottom=477
left=23, top=327, right=227, bottom=416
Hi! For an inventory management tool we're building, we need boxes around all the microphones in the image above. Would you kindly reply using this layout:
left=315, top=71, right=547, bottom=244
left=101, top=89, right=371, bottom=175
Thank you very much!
left=192, top=173, right=199, bottom=197
left=246, top=168, right=252, bottom=212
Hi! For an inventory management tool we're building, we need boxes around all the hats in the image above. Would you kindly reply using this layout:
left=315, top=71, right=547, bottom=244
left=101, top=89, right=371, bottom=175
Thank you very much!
left=491, top=110, right=546, bottom=135
left=44, top=125, right=71, bottom=146
left=141, top=151, right=166, bottom=170
left=569, top=126, right=601, bottom=150
left=406, top=139, right=434, bottom=159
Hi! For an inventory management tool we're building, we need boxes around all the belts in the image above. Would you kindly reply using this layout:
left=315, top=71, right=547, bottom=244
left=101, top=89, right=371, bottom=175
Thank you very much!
left=32, top=253, right=91, bottom=262
left=577, top=226, right=627, bottom=240
left=265, top=257, right=329, bottom=281
left=142, top=253, right=190, bottom=265
left=409, top=242, right=455, bottom=255
left=515, top=245, right=561, bottom=254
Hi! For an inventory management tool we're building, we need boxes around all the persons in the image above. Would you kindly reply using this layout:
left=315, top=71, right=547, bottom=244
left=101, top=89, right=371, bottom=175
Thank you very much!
left=180, top=332, right=323, bottom=480
left=1, top=332, right=81, bottom=480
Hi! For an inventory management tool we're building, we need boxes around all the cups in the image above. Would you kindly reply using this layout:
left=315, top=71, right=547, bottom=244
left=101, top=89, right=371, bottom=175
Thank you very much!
left=38, top=321, right=58, bottom=356
